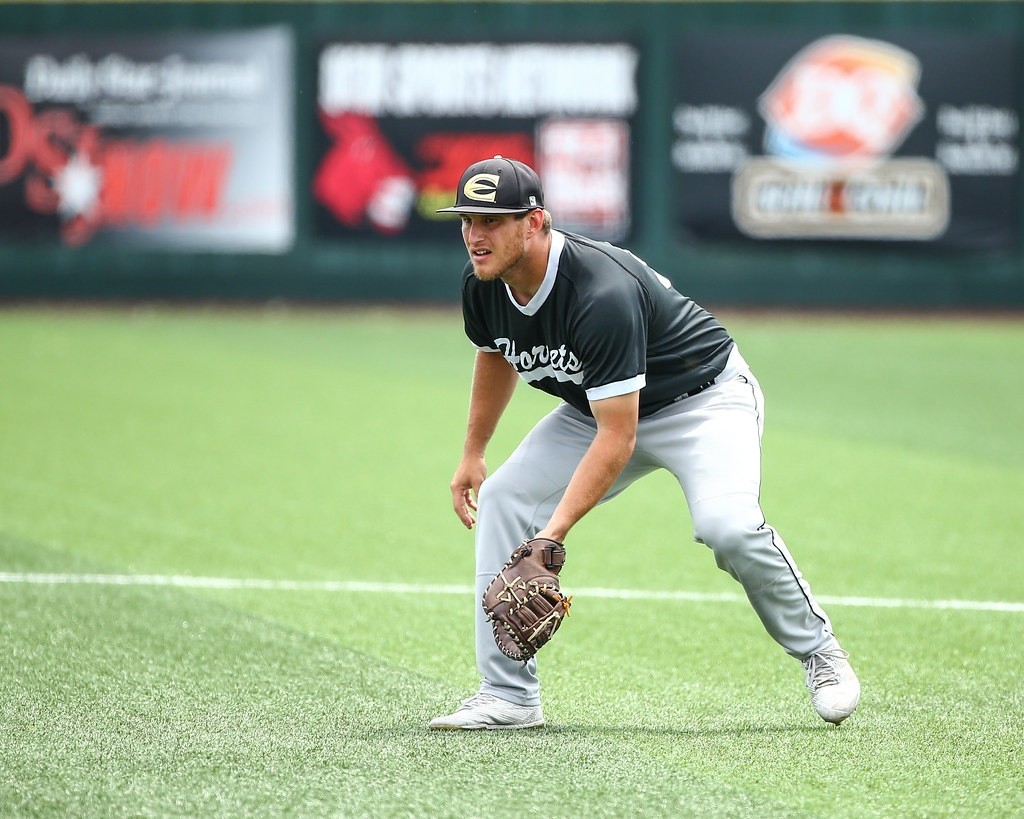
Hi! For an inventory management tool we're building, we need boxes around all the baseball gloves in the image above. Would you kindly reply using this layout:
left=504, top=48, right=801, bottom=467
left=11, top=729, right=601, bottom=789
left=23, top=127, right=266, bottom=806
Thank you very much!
left=480, top=536, right=575, bottom=670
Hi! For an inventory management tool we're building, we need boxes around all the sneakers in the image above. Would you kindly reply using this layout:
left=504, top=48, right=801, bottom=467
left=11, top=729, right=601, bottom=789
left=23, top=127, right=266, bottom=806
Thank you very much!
left=429, top=678, right=543, bottom=731
left=801, top=637, right=861, bottom=725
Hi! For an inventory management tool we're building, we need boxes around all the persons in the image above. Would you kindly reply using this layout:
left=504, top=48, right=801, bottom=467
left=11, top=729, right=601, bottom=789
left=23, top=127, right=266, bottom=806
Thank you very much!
left=427, top=157, right=861, bottom=729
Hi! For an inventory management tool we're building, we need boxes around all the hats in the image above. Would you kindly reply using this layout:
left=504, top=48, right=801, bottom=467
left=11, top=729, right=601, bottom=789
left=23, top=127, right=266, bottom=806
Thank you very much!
left=435, top=155, right=545, bottom=215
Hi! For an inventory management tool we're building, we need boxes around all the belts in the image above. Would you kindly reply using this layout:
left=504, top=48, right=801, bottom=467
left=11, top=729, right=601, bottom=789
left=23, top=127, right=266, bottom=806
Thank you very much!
left=643, top=378, right=714, bottom=418
